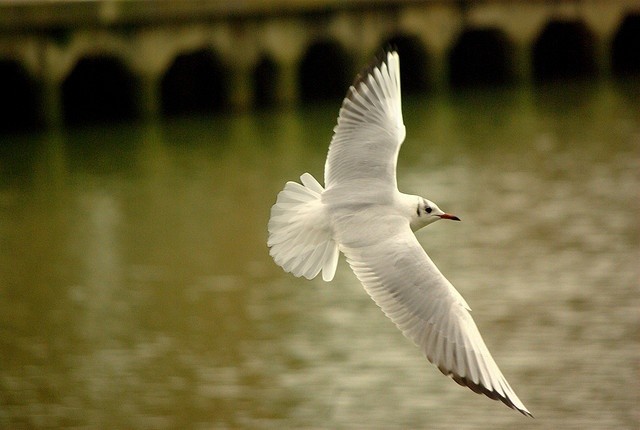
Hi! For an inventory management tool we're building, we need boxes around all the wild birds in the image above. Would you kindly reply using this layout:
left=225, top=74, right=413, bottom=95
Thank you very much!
left=267, top=50, right=532, bottom=419
